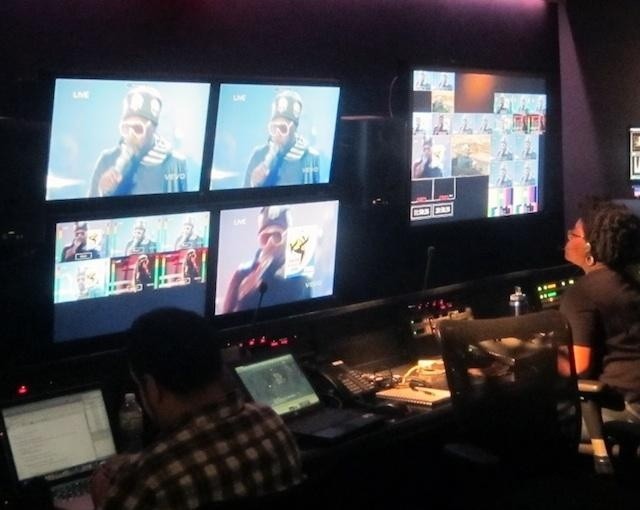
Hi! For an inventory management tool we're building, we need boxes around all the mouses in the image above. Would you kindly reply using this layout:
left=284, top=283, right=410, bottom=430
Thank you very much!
left=376, top=399, right=409, bottom=418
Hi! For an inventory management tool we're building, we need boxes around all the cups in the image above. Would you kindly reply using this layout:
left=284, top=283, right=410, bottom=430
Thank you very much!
left=374, top=367, right=391, bottom=390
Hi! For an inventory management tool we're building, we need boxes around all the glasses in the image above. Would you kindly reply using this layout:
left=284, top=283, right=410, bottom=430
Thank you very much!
left=133, top=232, right=142, bottom=236
left=567, top=230, right=584, bottom=242
left=75, top=232, right=85, bottom=238
left=268, top=121, right=295, bottom=136
left=119, top=121, right=151, bottom=139
left=257, top=233, right=284, bottom=248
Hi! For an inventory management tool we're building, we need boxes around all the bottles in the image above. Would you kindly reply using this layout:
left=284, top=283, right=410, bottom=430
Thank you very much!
left=509, top=286, right=528, bottom=318
left=120, top=393, right=143, bottom=453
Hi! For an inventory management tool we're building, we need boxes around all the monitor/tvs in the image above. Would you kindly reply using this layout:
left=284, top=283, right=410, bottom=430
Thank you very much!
left=41, top=75, right=217, bottom=208
left=47, top=202, right=215, bottom=356
left=406, top=65, right=548, bottom=230
left=203, top=77, right=345, bottom=202
left=208, top=190, right=344, bottom=330
left=627, top=125, right=640, bottom=183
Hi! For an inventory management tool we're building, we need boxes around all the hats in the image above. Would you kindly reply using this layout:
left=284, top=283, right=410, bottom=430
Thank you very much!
left=271, top=91, right=304, bottom=126
left=73, top=221, right=87, bottom=231
left=134, top=219, right=146, bottom=230
left=422, top=135, right=432, bottom=147
left=183, top=216, right=195, bottom=227
left=423, top=147, right=431, bottom=152
left=186, top=251, right=196, bottom=260
left=138, top=256, right=148, bottom=262
left=259, top=206, right=290, bottom=232
left=122, top=88, right=162, bottom=125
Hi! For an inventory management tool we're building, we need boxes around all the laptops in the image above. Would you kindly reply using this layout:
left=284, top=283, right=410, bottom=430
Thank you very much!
left=0, top=381, right=121, bottom=510
left=229, top=351, right=386, bottom=447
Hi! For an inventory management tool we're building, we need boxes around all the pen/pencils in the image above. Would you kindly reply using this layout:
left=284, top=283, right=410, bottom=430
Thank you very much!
left=411, top=386, right=438, bottom=396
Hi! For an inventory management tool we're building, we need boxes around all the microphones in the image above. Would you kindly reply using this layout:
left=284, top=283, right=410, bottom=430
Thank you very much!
left=240, top=280, right=269, bottom=360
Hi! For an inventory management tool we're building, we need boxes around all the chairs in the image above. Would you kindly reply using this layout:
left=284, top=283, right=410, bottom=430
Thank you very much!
left=440, top=310, right=580, bottom=496
left=581, top=419, right=640, bottom=509
left=196, top=472, right=361, bottom=510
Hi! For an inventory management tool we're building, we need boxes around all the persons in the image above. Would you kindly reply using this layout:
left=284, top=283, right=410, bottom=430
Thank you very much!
left=132, top=254, right=153, bottom=292
left=557, top=194, right=639, bottom=444
left=181, top=249, right=199, bottom=283
left=172, top=216, right=205, bottom=251
left=61, top=220, right=100, bottom=264
left=85, top=304, right=309, bottom=510
left=239, top=89, right=319, bottom=187
left=88, top=84, right=187, bottom=200
left=408, top=70, right=548, bottom=225
left=220, top=205, right=312, bottom=312
left=125, top=216, right=157, bottom=257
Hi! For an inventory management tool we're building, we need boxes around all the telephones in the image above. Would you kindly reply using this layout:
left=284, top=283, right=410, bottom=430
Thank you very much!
left=314, top=360, right=376, bottom=406
